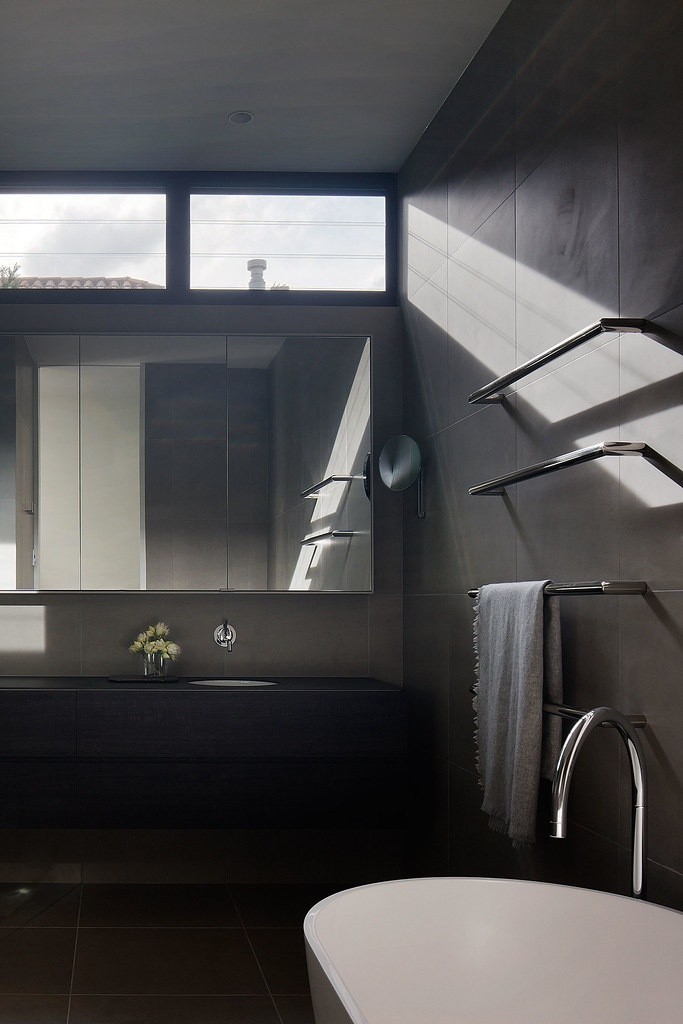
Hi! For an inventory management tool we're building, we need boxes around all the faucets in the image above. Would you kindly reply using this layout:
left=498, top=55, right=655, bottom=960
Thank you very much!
left=550, top=703, right=648, bottom=896
left=213, top=619, right=237, bottom=653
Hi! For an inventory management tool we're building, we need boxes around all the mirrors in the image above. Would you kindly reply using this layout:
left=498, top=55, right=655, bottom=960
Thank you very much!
left=379, top=434, right=422, bottom=491
left=0, top=330, right=376, bottom=597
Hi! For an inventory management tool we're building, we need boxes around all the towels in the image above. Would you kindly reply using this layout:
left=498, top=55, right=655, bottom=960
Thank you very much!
left=474, top=580, right=566, bottom=851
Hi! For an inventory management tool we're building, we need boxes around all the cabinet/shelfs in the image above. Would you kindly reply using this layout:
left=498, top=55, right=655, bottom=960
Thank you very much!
left=37, top=365, right=146, bottom=590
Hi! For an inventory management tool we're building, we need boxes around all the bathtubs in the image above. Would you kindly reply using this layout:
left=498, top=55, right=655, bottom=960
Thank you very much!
left=303, top=876, right=683, bottom=1023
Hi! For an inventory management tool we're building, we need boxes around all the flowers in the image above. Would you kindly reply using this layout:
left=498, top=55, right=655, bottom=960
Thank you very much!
left=128, top=623, right=182, bottom=671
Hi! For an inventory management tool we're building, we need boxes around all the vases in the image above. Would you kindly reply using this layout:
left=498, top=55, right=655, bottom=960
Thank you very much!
left=144, top=654, right=176, bottom=678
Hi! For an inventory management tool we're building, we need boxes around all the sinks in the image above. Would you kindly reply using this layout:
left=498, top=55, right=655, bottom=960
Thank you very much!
left=188, top=679, right=280, bottom=687
